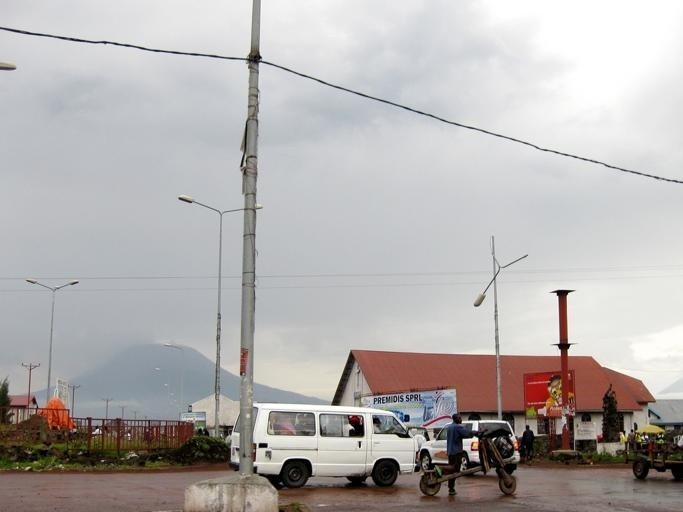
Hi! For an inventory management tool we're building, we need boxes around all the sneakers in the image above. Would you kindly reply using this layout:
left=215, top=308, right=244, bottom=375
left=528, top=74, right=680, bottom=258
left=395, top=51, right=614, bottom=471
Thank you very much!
left=449, top=492, right=456, bottom=495
left=434, top=465, right=442, bottom=477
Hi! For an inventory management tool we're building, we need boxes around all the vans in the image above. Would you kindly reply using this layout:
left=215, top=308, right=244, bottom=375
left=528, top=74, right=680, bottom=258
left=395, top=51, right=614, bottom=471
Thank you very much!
left=229, top=401, right=420, bottom=489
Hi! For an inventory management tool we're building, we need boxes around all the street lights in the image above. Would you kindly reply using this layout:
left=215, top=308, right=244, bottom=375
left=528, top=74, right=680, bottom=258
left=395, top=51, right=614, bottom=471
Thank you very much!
left=67, top=385, right=82, bottom=418
left=101, top=397, right=138, bottom=418
left=177, top=196, right=263, bottom=440
left=164, top=343, right=183, bottom=422
left=473, top=235, right=528, bottom=421
left=26, top=278, right=79, bottom=403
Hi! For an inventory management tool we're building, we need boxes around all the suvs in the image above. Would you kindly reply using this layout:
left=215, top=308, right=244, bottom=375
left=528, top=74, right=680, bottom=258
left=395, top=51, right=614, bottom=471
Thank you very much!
left=415, top=419, right=520, bottom=472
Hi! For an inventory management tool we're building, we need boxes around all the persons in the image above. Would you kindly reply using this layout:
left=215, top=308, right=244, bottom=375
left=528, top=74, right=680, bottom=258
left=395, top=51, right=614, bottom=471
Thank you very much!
left=521, top=425, right=536, bottom=465
left=619, top=428, right=650, bottom=454
left=91, top=426, right=100, bottom=438
left=124, top=429, right=134, bottom=441
left=372, top=417, right=391, bottom=434
left=273, top=413, right=361, bottom=438
left=146, top=428, right=154, bottom=443
left=657, top=432, right=665, bottom=443
left=196, top=427, right=209, bottom=436
left=70, top=424, right=78, bottom=432
left=446, top=414, right=479, bottom=497
left=226, top=428, right=233, bottom=466
